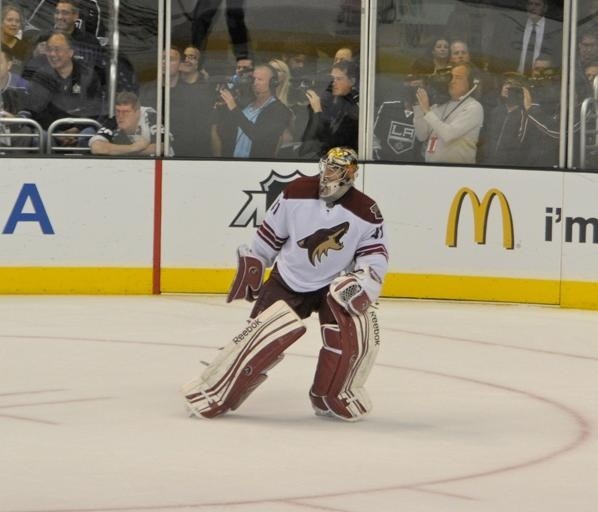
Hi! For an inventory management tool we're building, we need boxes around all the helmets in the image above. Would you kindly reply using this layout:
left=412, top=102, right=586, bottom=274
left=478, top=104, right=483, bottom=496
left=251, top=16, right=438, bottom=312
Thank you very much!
left=318, top=147, right=358, bottom=203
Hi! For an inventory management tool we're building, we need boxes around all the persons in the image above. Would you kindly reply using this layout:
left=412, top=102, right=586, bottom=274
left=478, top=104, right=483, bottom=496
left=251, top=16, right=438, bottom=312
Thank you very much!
left=184, top=147, right=389, bottom=421
left=1, top=2, right=598, bottom=173
